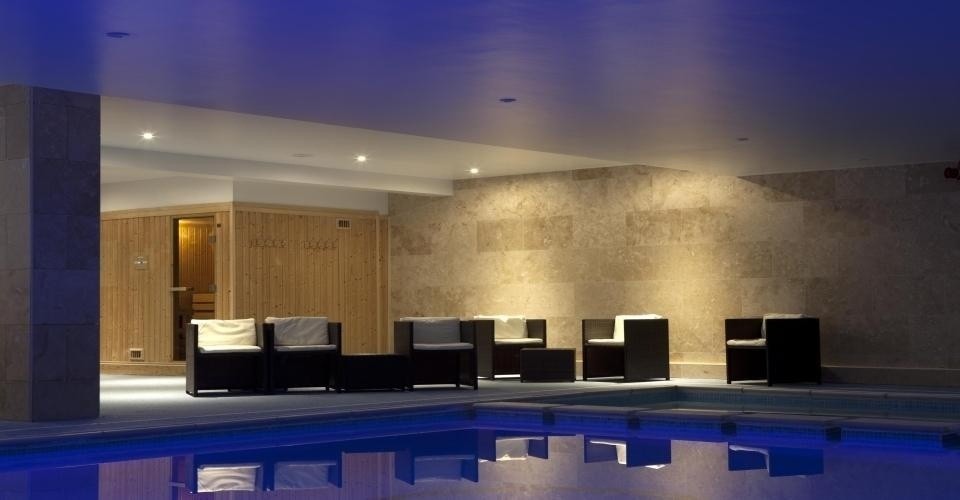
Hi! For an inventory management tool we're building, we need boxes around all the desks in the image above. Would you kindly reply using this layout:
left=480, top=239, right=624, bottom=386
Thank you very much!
left=519, top=348, right=576, bottom=383
left=342, top=352, right=404, bottom=391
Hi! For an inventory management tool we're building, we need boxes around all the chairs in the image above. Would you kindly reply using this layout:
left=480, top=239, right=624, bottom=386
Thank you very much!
left=394, top=317, right=478, bottom=390
left=469, top=315, right=546, bottom=379
left=725, top=314, right=821, bottom=387
left=264, top=316, right=341, bottom=394
left=582, top=313, right=670, bottom=381
left=185, top=318, right=267, bottom=396
